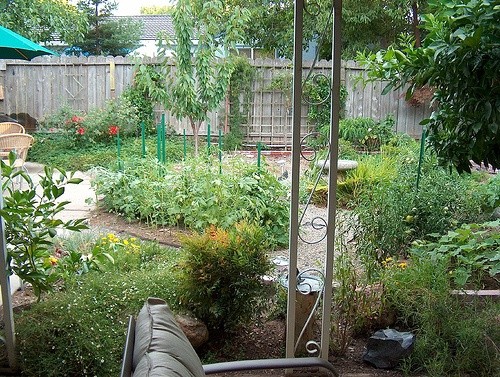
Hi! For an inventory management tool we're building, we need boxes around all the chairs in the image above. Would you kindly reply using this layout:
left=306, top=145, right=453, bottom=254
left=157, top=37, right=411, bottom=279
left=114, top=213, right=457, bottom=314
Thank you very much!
left=0, top=133, right=34, bottom=191
left=122, top=296, right=338, bottom=377
left=0, top=122, right=26, bottom=158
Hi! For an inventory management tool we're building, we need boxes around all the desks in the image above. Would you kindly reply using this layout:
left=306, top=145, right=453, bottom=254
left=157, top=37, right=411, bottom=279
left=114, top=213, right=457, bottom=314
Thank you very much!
left=316, top=160, right=357, bottom=183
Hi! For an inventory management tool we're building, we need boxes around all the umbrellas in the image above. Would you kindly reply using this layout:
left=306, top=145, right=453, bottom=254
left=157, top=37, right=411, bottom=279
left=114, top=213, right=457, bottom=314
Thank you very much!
left=0, top=25, right=60, bottom=62
